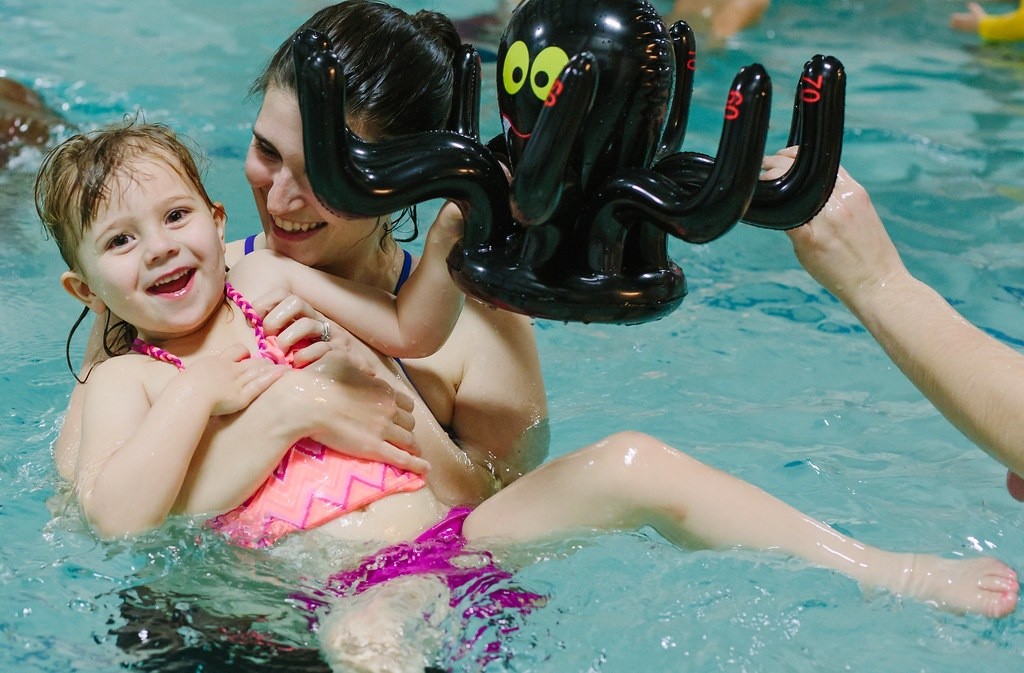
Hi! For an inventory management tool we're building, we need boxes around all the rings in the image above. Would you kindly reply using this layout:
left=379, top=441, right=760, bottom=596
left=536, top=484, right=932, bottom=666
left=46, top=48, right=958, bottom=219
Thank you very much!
left=316, top=319, right=331, bottom=342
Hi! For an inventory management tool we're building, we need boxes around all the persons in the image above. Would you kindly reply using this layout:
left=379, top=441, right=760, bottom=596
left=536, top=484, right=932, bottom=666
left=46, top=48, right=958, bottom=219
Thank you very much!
left=763, top=144, right=1024, bottom=504
left=949, top=1, right=1022, bottom=50
left=35, top=104, right=1019, bottom=620
left=54, top=0, right=554, bottom=522
left=648, top=1, right=770, bottom=34
